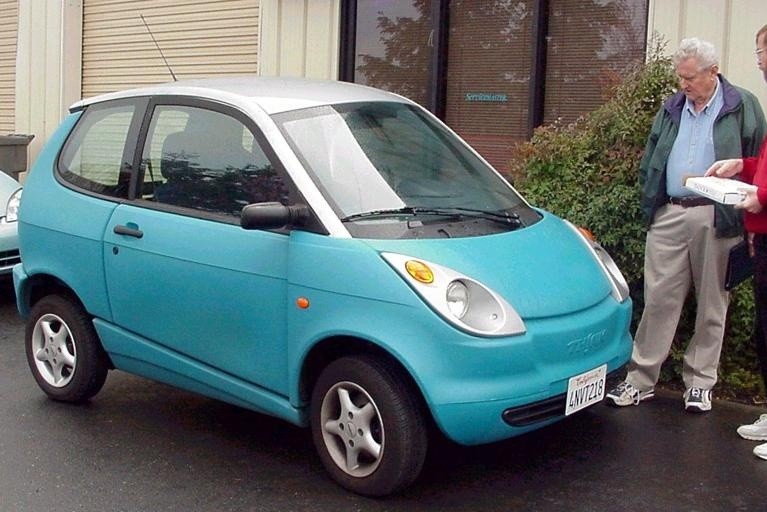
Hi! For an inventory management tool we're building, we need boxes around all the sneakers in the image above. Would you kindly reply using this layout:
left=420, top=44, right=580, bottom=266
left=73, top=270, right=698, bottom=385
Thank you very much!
left=683, top=387, right=713, bottom=413
left=737, top=414, right=767, bottom=440
left=755, top=444, right=767, bottom=460
left=605, top=380, right=657, bottom=408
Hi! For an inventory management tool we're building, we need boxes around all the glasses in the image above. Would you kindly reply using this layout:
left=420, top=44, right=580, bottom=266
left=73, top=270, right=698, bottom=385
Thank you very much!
left=751, top=47, right=766, bottom=65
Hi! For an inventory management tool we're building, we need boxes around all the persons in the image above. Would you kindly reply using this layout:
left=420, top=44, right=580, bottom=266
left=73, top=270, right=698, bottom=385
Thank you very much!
left=705, top=25, right=767, bottom=460
left=604, top=38, right=766, bottom=413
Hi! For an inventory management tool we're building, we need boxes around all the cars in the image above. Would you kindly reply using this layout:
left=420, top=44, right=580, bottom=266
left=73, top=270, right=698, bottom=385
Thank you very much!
left=13, top=76, right=635, bottom=498
left=0, top=169, right=24, bottom=278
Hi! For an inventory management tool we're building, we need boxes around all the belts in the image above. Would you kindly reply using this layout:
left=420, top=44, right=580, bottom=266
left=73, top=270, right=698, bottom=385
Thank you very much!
left=667, top=196, right=715, bottom=209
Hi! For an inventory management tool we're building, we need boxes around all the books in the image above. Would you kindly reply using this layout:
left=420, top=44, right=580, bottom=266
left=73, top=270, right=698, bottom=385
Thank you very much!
left=682, top=176, right=759, bottom=206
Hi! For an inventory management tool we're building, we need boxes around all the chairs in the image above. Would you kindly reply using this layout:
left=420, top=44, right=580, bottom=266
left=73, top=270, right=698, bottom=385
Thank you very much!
left=203, top=116, right=253, bottom=212
left=152, top=132, right=214, bottom=212
